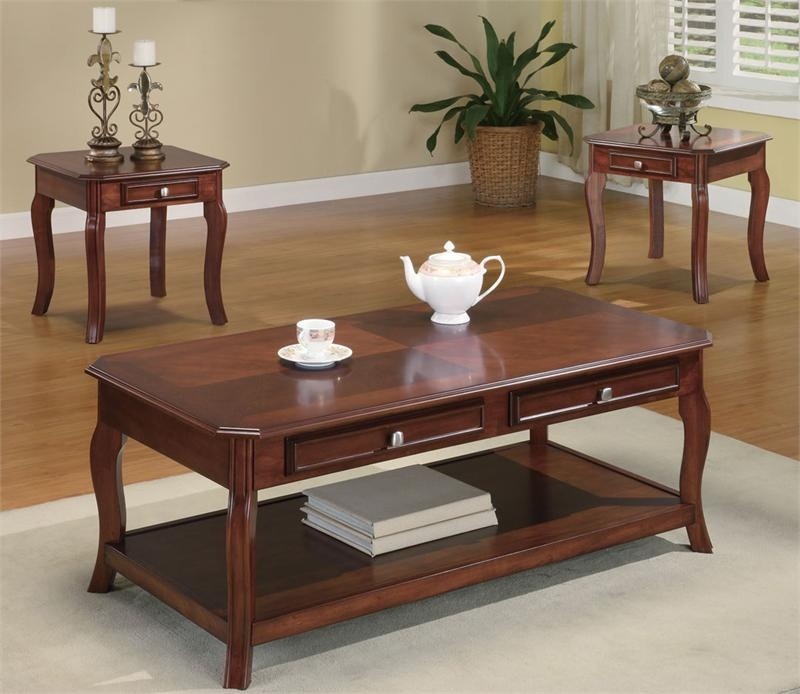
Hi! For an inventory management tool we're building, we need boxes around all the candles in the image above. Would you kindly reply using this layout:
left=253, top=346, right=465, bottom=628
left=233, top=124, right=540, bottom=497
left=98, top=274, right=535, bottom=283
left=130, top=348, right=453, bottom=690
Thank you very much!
left=134, top=39, right=156, bottom=65
left=93, top=7, right=117, bottom=33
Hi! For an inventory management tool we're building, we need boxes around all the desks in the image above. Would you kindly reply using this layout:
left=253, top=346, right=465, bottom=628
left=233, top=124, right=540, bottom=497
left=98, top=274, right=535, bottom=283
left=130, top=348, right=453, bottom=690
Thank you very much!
left=83, top=285, right=716, bottom=690
left=583, top=123, right=774, bottom=303
left=26, top=145, right=230, bottom=344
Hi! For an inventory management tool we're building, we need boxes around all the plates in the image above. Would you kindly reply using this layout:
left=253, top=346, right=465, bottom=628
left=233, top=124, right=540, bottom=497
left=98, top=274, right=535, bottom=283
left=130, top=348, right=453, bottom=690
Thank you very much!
left=277, top=344, right=353, bottom=366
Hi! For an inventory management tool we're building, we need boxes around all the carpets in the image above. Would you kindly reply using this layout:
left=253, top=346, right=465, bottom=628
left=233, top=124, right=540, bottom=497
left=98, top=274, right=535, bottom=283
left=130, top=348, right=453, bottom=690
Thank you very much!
left=1, top=405, right=800, bottom=694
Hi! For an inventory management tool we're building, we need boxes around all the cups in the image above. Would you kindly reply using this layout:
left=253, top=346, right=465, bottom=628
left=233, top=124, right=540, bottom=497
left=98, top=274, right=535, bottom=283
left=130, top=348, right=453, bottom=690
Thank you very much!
left=297, top=319, right=336, bottom=359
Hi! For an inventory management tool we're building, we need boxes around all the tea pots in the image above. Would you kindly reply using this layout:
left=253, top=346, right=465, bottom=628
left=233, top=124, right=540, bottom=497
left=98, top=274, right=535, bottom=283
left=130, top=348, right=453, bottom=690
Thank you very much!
left=399, top=240, right=506, bottom=324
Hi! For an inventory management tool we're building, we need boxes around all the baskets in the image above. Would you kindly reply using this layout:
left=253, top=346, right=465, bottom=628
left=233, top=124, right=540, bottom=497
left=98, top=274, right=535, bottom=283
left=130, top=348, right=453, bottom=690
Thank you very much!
left=463, top=118, right=545, bottom=208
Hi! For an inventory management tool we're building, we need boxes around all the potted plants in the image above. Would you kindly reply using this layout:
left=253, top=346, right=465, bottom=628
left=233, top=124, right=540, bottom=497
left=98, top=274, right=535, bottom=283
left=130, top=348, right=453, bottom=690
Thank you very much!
left=408, top=17, right=595, bottom=209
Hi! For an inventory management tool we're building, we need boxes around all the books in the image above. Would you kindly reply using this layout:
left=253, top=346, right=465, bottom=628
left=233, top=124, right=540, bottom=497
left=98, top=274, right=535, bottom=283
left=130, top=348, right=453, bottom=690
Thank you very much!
left=300, top=463, right=499, bottom=558
